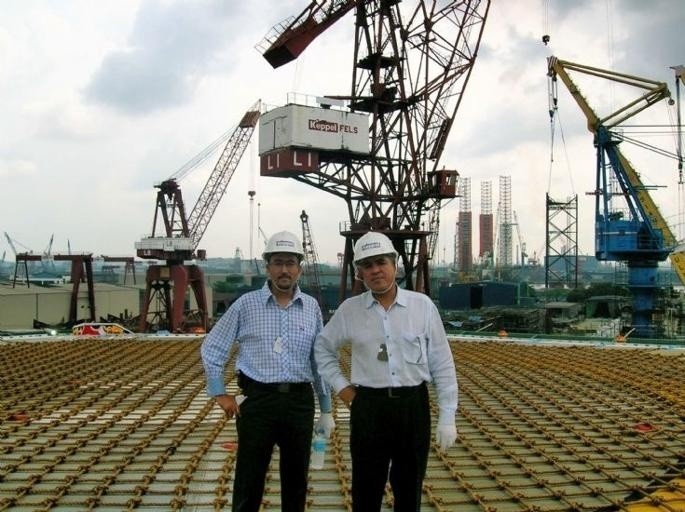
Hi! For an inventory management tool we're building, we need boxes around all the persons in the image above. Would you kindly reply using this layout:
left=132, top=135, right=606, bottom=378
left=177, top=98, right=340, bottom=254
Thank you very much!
left=200, top=231, right=335, bottom=512
left=313, top=231, right=459, bottom=512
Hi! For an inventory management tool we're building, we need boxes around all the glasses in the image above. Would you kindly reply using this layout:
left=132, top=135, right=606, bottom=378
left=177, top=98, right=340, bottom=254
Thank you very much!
left=268, top=261, right=299, bottom=272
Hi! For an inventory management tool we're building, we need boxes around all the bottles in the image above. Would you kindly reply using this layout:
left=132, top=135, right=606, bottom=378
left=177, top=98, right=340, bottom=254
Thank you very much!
left=310, top=430, right=327, bottom=470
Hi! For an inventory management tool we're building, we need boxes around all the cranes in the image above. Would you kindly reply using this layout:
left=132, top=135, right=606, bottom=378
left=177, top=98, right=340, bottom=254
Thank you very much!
left=2, top=228, right=58, bottom=273
left=541, top=34, right=685, bottom=338
left=132, top=98, right=262, bottom=334
left=257, top=0, right=494, bottom=333
left=299, top=209, right=331, bottom=326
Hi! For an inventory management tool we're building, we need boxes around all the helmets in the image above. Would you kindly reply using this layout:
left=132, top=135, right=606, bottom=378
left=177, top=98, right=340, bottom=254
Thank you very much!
left=262, top=230, right=305, bottom=260
left=354, top=232, right=398, bottom=265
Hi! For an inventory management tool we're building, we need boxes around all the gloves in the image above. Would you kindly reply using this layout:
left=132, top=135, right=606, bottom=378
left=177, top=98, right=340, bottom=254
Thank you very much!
left=436, top=423, right=457, bottom=453
left=315, top=413, right=336, bottom=439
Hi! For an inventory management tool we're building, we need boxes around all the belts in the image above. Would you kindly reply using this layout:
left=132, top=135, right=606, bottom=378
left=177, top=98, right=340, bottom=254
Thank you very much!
left=237, top=372, right=313, bottom=394
left=357, top=385, right=427, bottom=398
